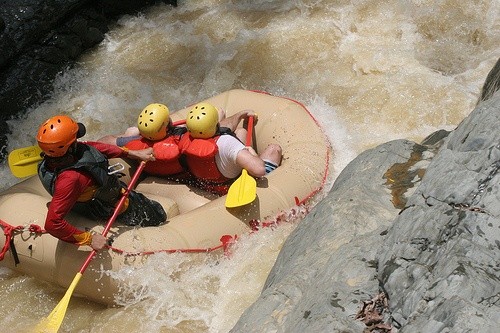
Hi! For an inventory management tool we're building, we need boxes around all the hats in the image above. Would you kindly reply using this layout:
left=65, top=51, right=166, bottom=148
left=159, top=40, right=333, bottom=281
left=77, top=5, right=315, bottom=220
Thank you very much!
left=76, top=123, right=86, bottom=138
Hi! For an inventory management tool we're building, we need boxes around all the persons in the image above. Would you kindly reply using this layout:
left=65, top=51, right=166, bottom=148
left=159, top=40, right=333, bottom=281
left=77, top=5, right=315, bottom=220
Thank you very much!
left=34, top=112, right=168, bottom=252
left=179, top=103, right=283, bottom=196
left=95, top=103, right=225, bottom=178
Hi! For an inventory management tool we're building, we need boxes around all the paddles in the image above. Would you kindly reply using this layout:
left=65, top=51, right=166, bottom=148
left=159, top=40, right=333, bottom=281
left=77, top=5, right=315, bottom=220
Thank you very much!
left=224, top=116, right=258, bottom=210
left=8, top=119, right=187, bottom=179
left=36, top=153, right=151, bottom=333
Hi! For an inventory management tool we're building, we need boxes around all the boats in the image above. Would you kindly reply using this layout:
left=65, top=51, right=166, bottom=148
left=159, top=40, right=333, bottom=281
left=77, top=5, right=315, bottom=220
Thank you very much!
left=0, top=88, right=337, bottom=307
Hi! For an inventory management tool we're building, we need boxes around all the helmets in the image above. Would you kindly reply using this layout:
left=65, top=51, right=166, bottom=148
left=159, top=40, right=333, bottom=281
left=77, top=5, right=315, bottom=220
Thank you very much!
left=185, top=102, right=220, bottom=139
left=137, top=103, right=170, bottom=141
left=37, top=116, right=80, bottom=157
